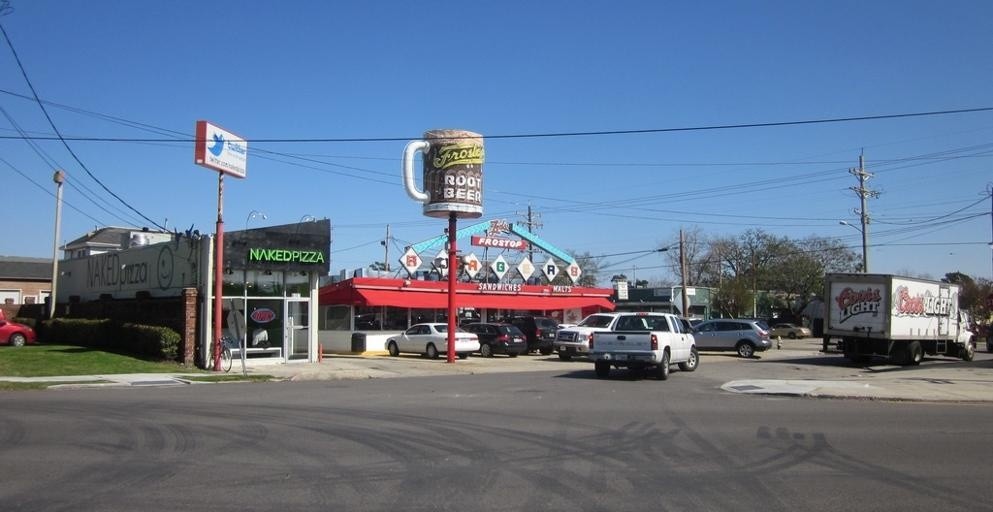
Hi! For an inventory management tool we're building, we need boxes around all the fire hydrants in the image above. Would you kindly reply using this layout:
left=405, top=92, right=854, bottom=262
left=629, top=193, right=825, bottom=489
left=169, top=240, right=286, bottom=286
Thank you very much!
left=776, top=335, right=783, bottom=349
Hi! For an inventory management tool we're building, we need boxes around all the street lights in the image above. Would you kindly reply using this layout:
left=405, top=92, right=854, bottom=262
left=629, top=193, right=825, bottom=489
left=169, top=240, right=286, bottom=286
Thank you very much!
left=838, top=220, right=869, bottom=272
left=50, top=169, right=66, bottom=320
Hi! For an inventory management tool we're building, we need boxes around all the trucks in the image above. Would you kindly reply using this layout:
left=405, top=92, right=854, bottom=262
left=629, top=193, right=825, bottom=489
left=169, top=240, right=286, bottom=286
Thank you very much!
left=823, top=272, right=974, bottom=367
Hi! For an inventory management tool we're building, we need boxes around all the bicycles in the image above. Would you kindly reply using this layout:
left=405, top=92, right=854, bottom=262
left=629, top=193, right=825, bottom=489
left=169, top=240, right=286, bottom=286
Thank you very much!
left=194, top=334, right=236, bottom=373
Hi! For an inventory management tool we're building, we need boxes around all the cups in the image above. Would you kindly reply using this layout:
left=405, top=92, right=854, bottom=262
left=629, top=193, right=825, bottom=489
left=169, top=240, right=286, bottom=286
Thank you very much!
left=401, top=130, right=483, bottom=219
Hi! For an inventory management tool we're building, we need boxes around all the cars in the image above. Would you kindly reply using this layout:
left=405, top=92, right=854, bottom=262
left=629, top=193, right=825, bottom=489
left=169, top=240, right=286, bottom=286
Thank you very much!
left=985, top=321, right=993, bottom=352
left=770, top=322, right=814, bottom=339
left=0, top=308, right=36, bottom=346
left=333, top=313, right=386, bottom=331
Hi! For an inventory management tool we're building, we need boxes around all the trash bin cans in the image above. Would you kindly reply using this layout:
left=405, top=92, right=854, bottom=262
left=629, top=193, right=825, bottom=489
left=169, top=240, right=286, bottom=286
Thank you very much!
left=352, top=333, right=366, bottom=352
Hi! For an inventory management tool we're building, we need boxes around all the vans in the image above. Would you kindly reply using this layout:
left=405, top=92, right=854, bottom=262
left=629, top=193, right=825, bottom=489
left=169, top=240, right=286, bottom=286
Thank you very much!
left=692, top=318, right=772, bottom=359
